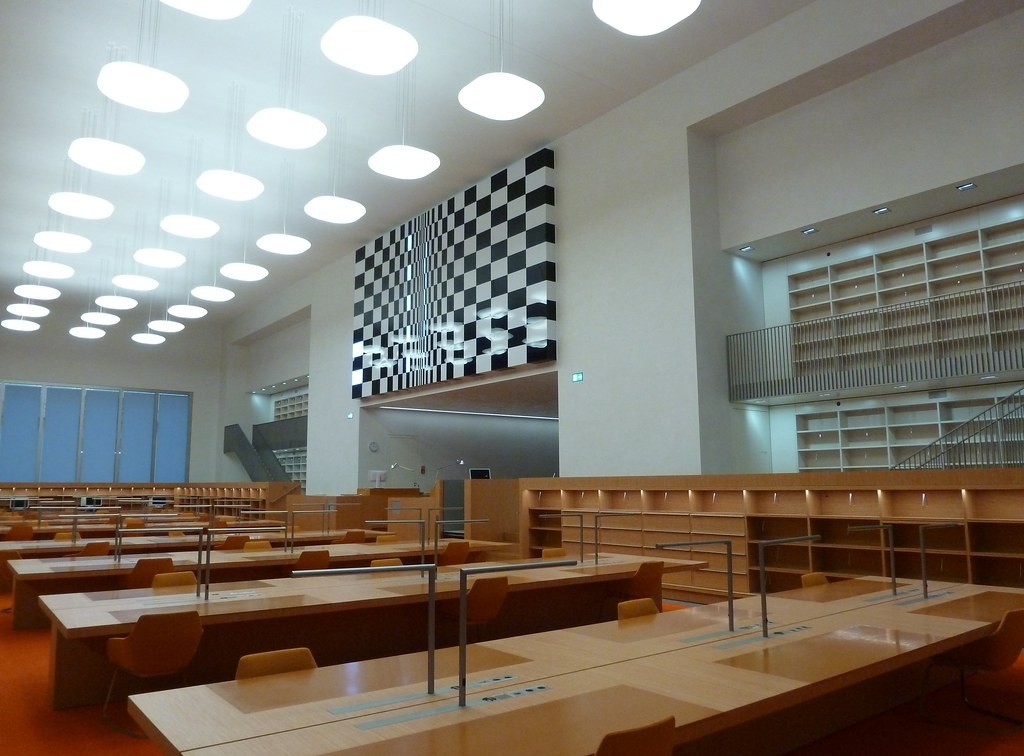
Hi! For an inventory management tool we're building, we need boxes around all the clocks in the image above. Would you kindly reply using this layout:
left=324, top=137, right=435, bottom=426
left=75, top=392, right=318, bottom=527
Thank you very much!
left=369, top=442, right=379, bottom=453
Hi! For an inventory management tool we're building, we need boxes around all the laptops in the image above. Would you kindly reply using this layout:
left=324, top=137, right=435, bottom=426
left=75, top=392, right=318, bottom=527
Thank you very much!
left=468, top=468, right=492, bottom=479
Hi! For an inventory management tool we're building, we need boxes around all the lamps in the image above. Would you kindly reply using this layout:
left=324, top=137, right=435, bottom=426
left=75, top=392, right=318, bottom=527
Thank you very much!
left=591, top=0, right=702, bottom=38
left=391, top=462, right=415, bottom=473
left=1, top=1, right=441, bottom=345
left=458, top=0, right=546, bottom=120
left=436, top=458, right=465, bottom=468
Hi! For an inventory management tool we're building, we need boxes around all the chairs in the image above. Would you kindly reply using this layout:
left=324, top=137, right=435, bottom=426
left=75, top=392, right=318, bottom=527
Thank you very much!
left=542, top=547, right=566, bottom=558
left=214, top=535, right=250, bottom=549
left=117, top=558, right=175, bottom=591
left=102, top=610, right=205, bottom=738
left=370, top=558, right=403, bottom=566
left=376, top=534, right=397, bottom=542
left=920, top=607, right=1024, bottom=735
left=331, top=530, right=367, bottom=543
left=244, top=540, right=273, bottom=550
left=3, top=524, right=35, bottom=542
left=435, top=576, right=509, bottom=650
left=597, top=560, right=664, bottom=622
left=293, top=549, right=331, bottom=573
left=124, top=522, right=145, bottom=537
left=800, top=569, right=834, bottom=589
left=65, top=542, right=110, bottom=557
left=617, top=597, right=659, bottom=619
left=54, top=532, right=81, bottom=540
left=437, top=541, right=470, bottom=566
left=152, top=572, right=199, bottom=588
left=594, top=716, right=675, bottom=756
left=235, top=648, right=319, bottom=680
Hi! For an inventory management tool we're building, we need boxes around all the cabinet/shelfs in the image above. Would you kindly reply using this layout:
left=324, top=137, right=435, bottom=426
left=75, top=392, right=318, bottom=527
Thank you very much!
left=521, top=467, right=1024, bottom=602
left=274, top=394, right=308, bottom=492
left=795, top=394, right=1024, bottom=472
left=176, top=482, right=301, bottom=519
left=787, top=217, right=1024, bottom=377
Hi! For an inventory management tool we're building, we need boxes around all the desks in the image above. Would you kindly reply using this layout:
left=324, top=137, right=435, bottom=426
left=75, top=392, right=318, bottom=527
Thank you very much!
left=0, top=510, right=1024, bottom=756
